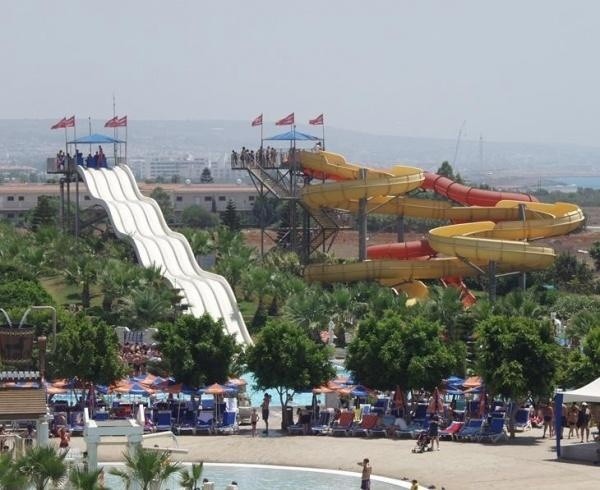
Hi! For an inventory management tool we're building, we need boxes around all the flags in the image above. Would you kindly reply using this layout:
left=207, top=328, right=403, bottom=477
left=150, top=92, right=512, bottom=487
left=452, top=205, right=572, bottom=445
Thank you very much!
left=111, top=115, right=127, bottom=126
left=64, top=115, right=74, bottom=127
left=51, top=116, right=66, bottom=129
left=252, top=115, right=261, bottom=127
left=309, top=113, right=323, bottom=125
left=104, top=116, right=118, bottom=127
left=276, top=112, right=294, bottom=125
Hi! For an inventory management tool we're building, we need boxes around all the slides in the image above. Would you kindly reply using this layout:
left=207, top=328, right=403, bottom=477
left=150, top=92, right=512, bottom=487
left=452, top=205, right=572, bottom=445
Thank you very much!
left=77, top=162, right=255, bottom=351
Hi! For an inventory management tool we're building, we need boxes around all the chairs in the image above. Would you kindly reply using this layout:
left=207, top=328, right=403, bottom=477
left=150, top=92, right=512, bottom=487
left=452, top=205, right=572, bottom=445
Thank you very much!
left=49, top=399, right=258, bottom=438
left=288, top=399, right=533, bottom=447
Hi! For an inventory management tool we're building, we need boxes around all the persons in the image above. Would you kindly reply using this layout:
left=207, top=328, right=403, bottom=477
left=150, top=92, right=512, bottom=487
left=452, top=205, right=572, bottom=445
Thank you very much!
left=23, top=425, right=36, bottom=438
left=357, top=458, right=372, bottom=490
left=411, top=480, right=419, bottom=490
left=58, top=427, right=72, bottom=456
left=260, top=393, right=270, bottom=433
left=251, top=408, right=260, bottom=437
left=427, top=411, right=440, bottom=450
left=540, top=400, right=592, bottom=443
left=56, top=145, right=106, bottom=172
left=83, top=451, right=88, bottom=473
left=116, top=340, right=164, bottom=377
left=231, top=142, right=323, bottom=168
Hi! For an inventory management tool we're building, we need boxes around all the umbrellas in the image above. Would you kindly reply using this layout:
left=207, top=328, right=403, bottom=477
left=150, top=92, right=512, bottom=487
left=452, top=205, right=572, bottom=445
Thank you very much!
left=424, top=375, right=490, bottom=420
left=296, top=374, right=377, bottom=408
left=43, top=371, right=247, bottom=425
left=391, top=384, right=403, bottom=416
